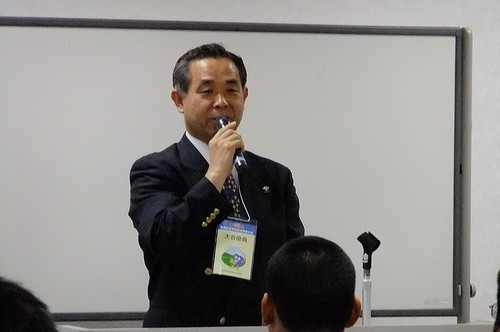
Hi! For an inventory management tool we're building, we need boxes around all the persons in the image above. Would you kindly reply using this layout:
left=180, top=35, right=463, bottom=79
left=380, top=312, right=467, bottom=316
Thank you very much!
left=0, top=272, right=57, bottom=332
left=261, top=235, right=361, bottom=332
left=128, top=44, right=306, bottom=328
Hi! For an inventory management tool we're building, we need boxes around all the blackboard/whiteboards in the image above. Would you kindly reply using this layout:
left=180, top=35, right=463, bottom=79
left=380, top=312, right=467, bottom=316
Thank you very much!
left=0, top=15, right=473, bottom=324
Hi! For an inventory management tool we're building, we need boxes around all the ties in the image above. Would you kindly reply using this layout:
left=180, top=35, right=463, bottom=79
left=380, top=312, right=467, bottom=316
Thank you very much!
left=224, top=172, right=246, bottom=222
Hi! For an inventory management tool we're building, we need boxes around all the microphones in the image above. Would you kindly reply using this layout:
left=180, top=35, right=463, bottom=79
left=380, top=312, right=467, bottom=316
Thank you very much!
left=214, top=115, right=248, bottom=174
